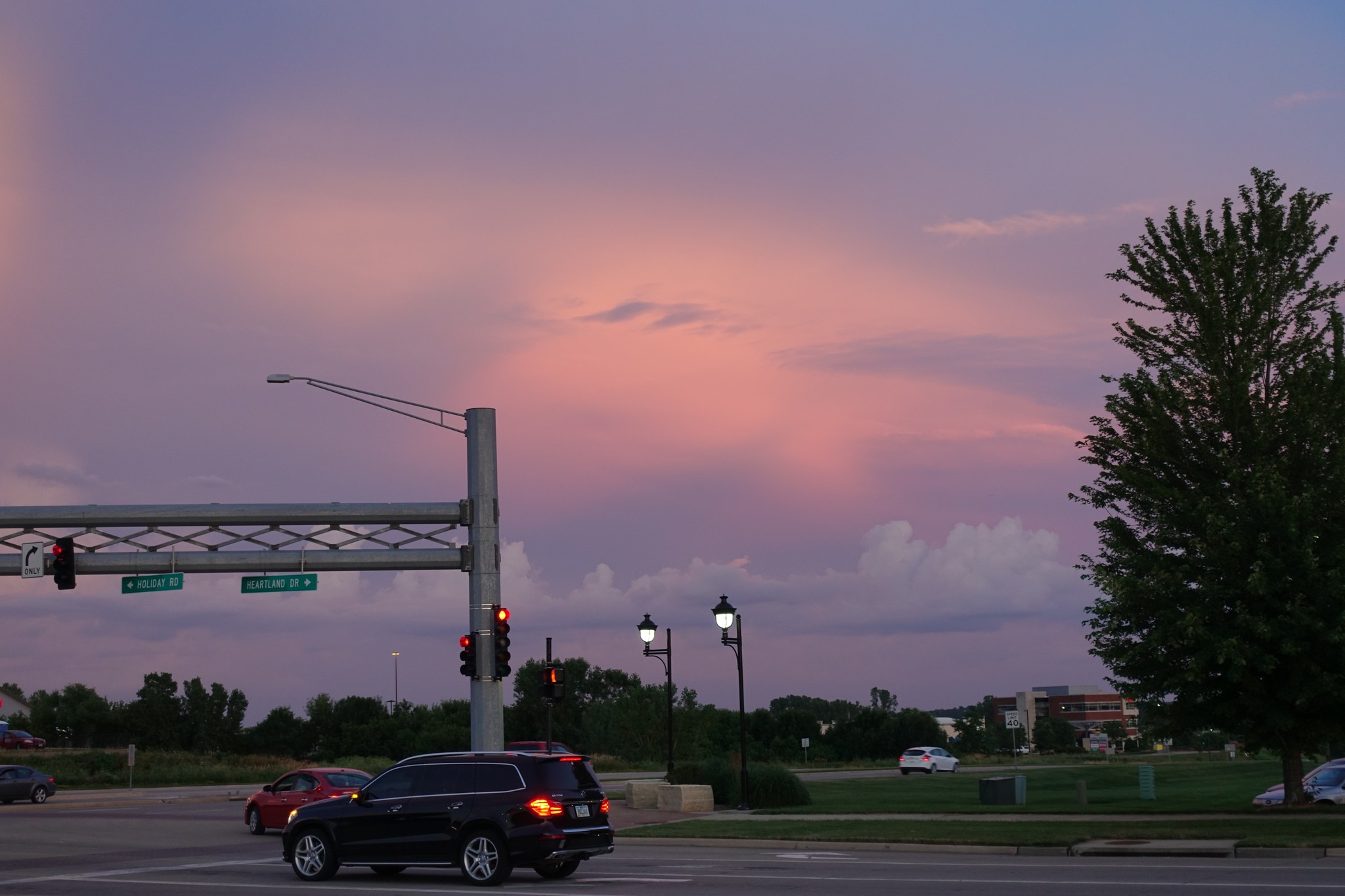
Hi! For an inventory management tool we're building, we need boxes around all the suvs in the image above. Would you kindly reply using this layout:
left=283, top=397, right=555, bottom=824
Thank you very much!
left=508, top=740, right=575, bottom=755
left=281, top=749, right=616, bottom=887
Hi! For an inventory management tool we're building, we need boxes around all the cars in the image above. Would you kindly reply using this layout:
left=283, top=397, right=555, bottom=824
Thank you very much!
left=0, top=729, right=46, bottom=750
left=1011, top=744, right=1031, bottom=755
left=244, top=767, right=373, bottom=835
left=899, top=746, right=960, bottom=776
left=1252, top=757, right=1345, bottom=808
left=0, top=764, right=56, bottom=804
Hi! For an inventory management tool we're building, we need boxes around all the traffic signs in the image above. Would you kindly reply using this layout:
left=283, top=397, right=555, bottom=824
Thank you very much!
left=241, top=573, right=317, bottom=593
left=121, top=572, right=183, bottom=594
left=21, top=541, right=44, bottom=578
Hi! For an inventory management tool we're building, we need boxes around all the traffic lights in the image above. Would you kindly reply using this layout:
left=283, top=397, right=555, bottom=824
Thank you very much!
left=492, top=607, right=511, bottom=677
left=52, top=537, right=75, bottom=590
left=550, top=668, right=564, bottom=685
left=460, top=634, right=477, bottom=676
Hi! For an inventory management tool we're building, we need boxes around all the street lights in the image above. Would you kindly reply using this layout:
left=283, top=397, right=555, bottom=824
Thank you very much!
left=710, top=593, right=755, bottom=810
left=266, top=373, right=506, bottom=753
left=386, top=700, right=396, bottom=715
left=391, top=652, right=400, bottom=705
left=636, top=612, right=674, bottom=785
left=1061, top=706, right=1065, bottom=753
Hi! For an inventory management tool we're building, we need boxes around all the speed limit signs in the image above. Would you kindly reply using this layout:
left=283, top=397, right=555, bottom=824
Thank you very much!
left=1005, top=710, right=1020, bottom=730
